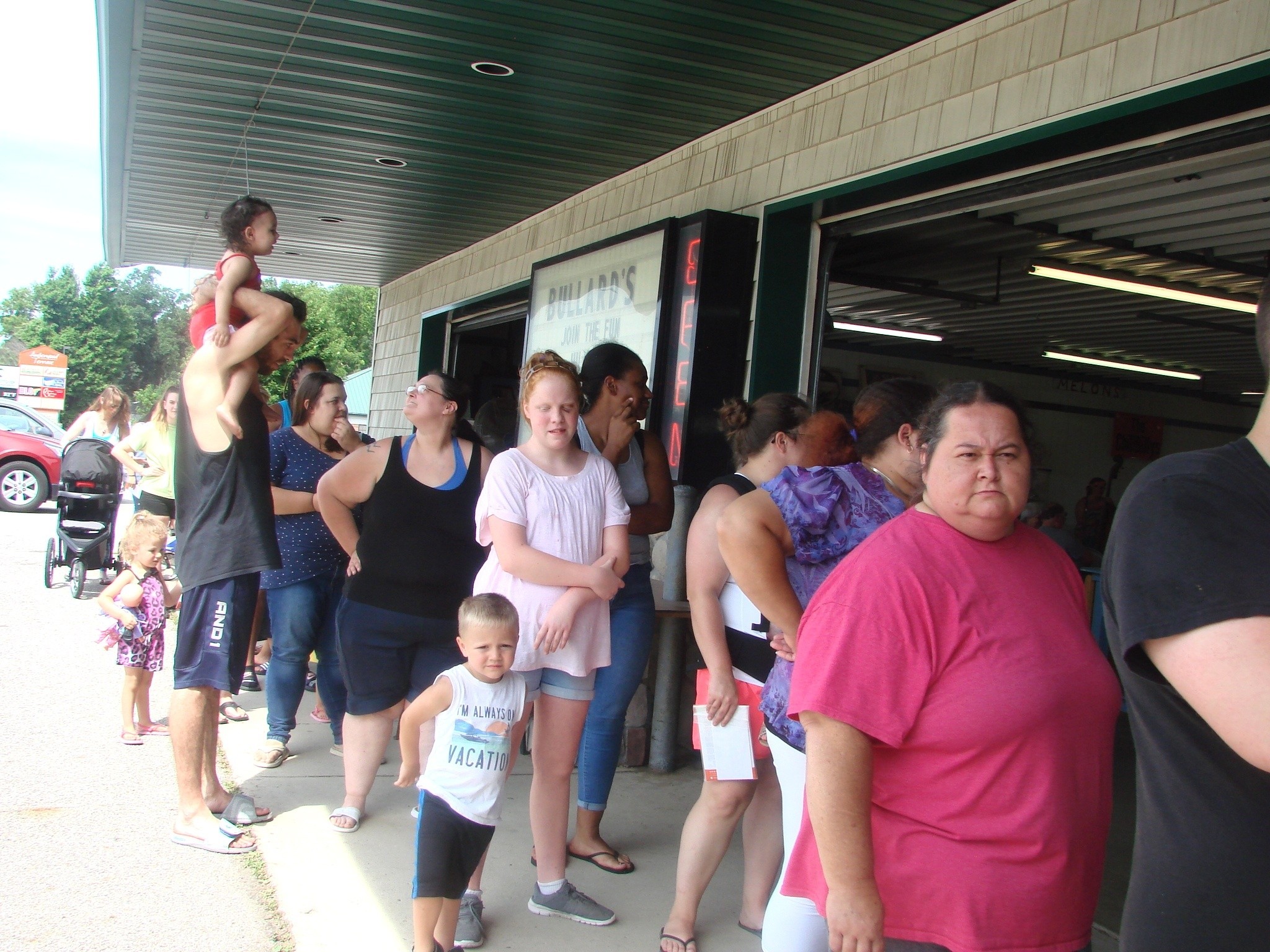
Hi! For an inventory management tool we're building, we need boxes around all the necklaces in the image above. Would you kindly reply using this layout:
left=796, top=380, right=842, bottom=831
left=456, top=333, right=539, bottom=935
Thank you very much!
left=863, top=457, right=913, bottom=501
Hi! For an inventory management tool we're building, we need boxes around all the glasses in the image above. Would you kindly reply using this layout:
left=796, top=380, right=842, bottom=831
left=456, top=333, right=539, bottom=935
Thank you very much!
left=405, top=385, right=452, bottom=400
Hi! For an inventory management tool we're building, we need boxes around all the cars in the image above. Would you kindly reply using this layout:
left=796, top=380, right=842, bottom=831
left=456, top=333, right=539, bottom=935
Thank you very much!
left=0, top=398, right=82, bottom=513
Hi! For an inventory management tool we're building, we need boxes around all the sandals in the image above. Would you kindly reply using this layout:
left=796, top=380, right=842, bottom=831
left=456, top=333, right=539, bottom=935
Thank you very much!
left=120, top=726, right=144, bottom=745
left=310, top=705, right=331, bottom=723
left=137, top=721, right=170, bottom=736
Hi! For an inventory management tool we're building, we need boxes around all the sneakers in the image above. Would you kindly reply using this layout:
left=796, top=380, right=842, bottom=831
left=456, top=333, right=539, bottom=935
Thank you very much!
left=454, top=894, right=488, bottom=948
left=528, top=879, right=615, bottom=926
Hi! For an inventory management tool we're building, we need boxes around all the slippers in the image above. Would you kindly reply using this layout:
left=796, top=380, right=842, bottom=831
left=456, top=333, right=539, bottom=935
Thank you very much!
left=212, top=794, right=273, bottom=824
left=410, top=807, right=421, bottom=818
left=304, top=671, right=317, bottom=692
left=217, top=702, right=249, bottom=724
left=531, top=843, right=569, bottom=869
left=170, top=817, right=257, bottom=854
left=659, top=927, right=696, bottom=952
left=330, top=745, right=388, bottom=764
left=567, top=842, right=635, bottom=874
left=254, top=661, right=270, bottom=675
left=328, top=806, right=367, bottom=832
left=239, top=670, right=261, bottom=691
left=737, top=921, right=762, bottom=939
left=251, top=745, right=290, bottom=768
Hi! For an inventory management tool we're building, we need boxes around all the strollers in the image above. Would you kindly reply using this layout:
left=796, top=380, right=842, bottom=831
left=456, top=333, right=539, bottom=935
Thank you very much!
left=44, top=438, right=124, bottom=599
left=159, top=531, right=178, bottom=582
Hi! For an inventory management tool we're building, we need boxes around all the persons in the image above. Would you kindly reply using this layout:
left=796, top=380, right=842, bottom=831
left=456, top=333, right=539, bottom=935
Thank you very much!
left=395, top=593, right=527, bottom=952
left=531, top=342, right=675, bottom=873
left=718, top=376, right=934, bottom=945
left=448, top=350, right=632, bottom=948
left=185, top=195, right=279, bottom=438
left=760, top=382, right=1121, bottom=952
left=1020, top=473, right=1115, bottom=569
left=165, top=286, right=307, bottom=855
left=258, top=371, right=374, bottom=768
left=659, top=392, right=814, bottom=952
left=60, top=386, right=136, bottom=585
left=109, top=356, right=325, bottom=693
left=1100, top=286, right=1269, bottom=952
left=315, top=373, right=494, bottom=834
left=95, top=507, right=170, bottom=746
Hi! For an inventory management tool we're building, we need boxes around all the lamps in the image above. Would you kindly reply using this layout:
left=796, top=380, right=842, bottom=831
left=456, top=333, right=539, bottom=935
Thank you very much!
left=1021, top=257, right=1259, bottom=317
left=833, top=316, right=946, bottom=343
left=1039, top=345, right=1205, bottom=388
